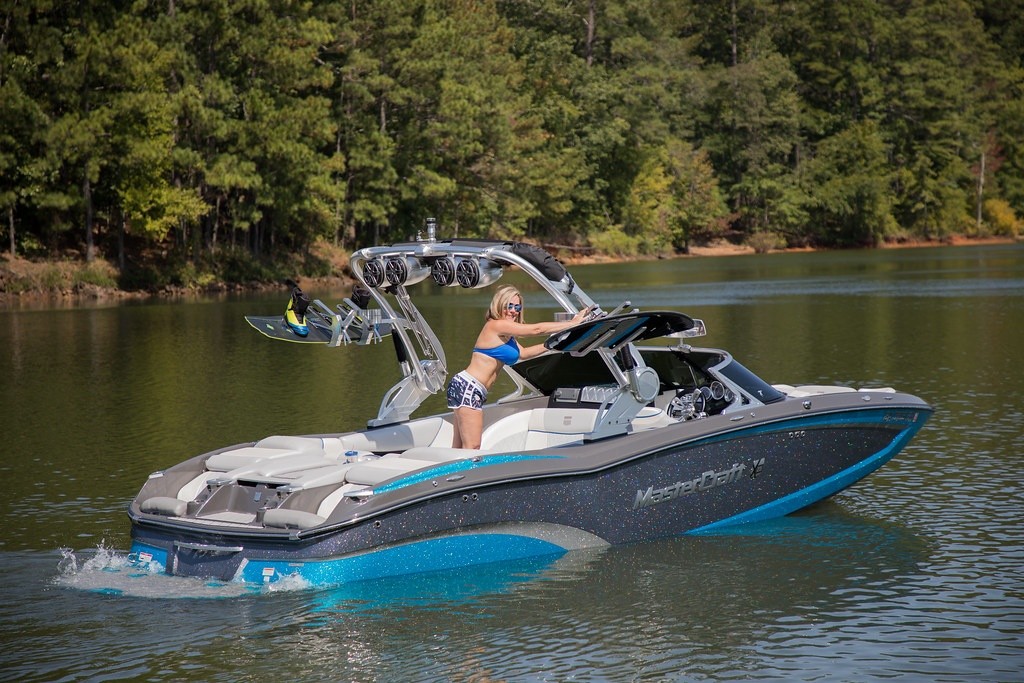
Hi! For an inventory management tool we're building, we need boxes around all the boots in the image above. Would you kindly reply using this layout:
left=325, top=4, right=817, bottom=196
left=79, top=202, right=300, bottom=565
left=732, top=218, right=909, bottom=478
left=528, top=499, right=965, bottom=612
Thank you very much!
left=346, top=286, right=380, bottom=331
left=285, top=287, right=310, bottom=337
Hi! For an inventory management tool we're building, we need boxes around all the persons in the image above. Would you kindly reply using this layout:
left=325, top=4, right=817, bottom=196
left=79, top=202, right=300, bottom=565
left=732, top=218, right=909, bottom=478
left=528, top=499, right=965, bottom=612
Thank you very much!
left=447, top=283, right=594, bottom=450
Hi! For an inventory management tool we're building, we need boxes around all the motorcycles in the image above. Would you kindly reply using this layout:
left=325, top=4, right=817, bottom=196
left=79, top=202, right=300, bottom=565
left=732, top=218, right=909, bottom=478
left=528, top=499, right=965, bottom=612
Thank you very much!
left=86, top=216, right=936, bottom=630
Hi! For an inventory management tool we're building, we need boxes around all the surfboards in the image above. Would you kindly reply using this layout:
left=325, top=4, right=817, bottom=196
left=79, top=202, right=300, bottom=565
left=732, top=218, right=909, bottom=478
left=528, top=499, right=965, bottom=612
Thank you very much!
left=542, top=309, right=695, bottom=353
left=245, top=310, right=410, bottom=344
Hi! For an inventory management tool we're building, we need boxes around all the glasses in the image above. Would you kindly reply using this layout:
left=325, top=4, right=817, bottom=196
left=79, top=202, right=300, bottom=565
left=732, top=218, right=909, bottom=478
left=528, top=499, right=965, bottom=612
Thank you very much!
left=507, top=303, right=522, bottom=311
